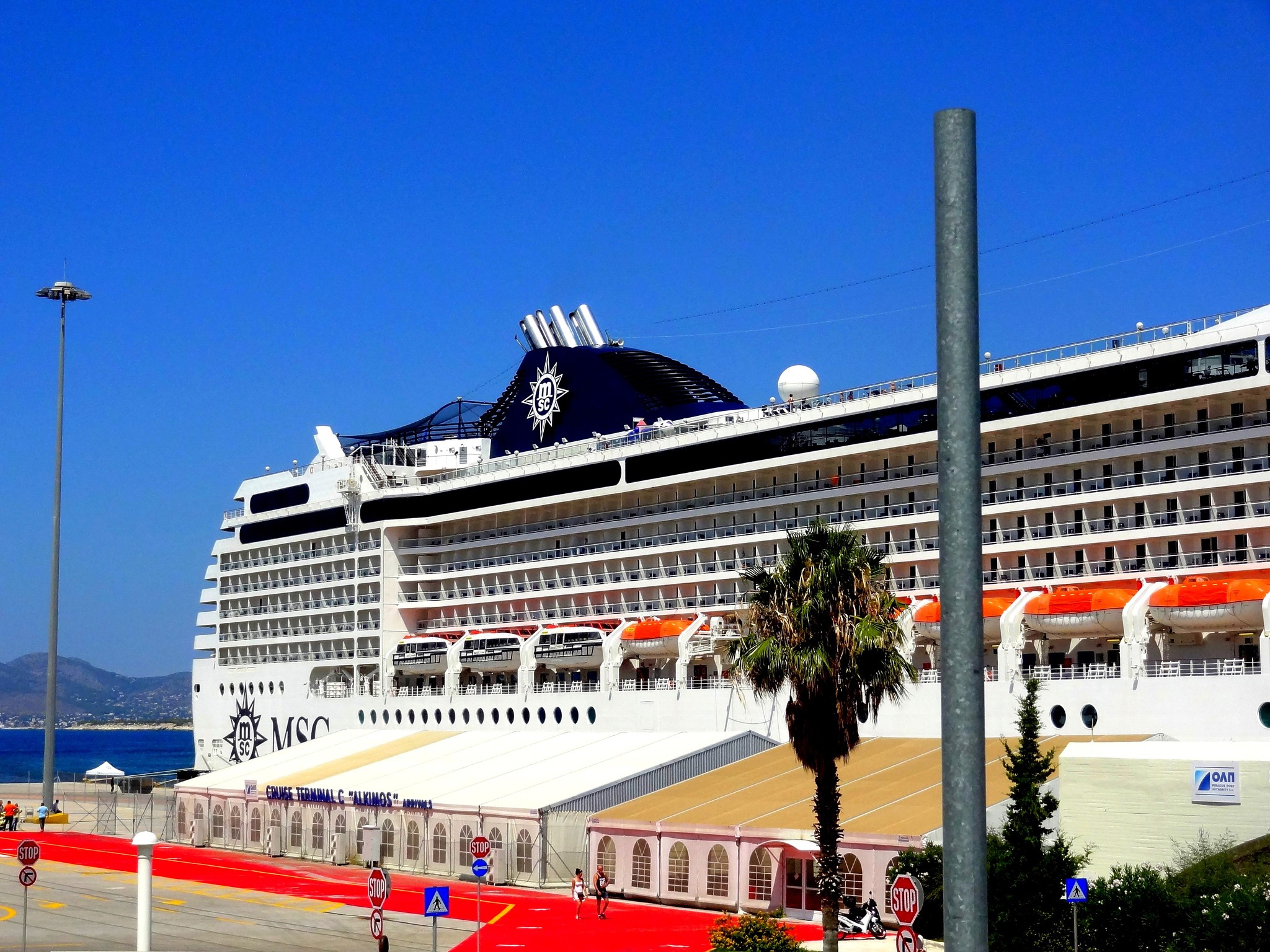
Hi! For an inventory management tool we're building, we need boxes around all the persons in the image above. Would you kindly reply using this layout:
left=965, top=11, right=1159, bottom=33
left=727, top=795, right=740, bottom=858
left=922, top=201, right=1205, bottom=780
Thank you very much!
left=797, top=398, right=821, bottom=410
left=827, top=394, right=832, bottom=405
left=571, top=868, right=588, bottom=920
left=11, top=803, right=22, bottom=831
left=111, top=777, right=114, bottom=794
left=37, top=803, right=49, bottom=832
left=593, top=865, right=610, bottom=920
left=840, top=391, right=844, bottom=403
left=886, top=383, right=896, bottom=393
left=53, top=799, right=62, bottom=814
left=0, top=800, right=4, bottom=831
left=787, top=394, right=794, bottom=413
left=870, top=390, right=876, bottom=398
left=3, top=801, right=18, bottom=832
left=847, top=391, right=855, bottom=401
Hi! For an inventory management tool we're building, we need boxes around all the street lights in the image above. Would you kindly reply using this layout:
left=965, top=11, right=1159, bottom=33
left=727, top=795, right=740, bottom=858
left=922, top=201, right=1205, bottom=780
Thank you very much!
left=35, top=259, right=94, bottom=820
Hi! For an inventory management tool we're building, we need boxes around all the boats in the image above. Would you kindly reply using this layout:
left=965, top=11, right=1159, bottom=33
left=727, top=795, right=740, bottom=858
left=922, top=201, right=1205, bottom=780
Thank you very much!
left=619, top=617, right=710, bottom=654
left=1026, top=585, right=1142, bottom=636
left=23, top=812, right=69, bottom=825
left=913, top=589, right=1020, bottom=642
left=868, top=597, right=911, bottom=620
left=459, top=633, right=525, bottom=674
left=392, top=636, right=453, bottom=675
left=1147, top=572, right=1270, bottom=634
left=534, top=626, right=607, bottom=668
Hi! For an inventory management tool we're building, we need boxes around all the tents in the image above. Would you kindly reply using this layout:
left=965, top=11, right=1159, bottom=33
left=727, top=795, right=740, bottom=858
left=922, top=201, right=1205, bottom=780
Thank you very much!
left=85, top=761, right=125, bottom=793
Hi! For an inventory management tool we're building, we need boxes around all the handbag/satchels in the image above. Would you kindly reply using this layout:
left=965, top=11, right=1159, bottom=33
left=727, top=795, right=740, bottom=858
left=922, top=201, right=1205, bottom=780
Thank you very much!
left=578, top=892, right=583, bottom=898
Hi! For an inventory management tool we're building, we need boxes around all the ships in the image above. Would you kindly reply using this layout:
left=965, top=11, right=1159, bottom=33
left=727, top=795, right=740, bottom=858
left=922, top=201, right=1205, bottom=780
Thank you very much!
left=177, top=301, right=1270, bottom=789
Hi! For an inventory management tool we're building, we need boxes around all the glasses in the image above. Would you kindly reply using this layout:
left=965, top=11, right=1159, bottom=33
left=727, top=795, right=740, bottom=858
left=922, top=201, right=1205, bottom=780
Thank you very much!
left=599, top=868, right=603, bottom=870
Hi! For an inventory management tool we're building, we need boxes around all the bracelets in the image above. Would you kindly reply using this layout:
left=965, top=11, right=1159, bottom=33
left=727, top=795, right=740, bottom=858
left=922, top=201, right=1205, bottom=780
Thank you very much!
left=605, top=884, right=608, bottom=887
left=597, top=890, right=599, bottom=892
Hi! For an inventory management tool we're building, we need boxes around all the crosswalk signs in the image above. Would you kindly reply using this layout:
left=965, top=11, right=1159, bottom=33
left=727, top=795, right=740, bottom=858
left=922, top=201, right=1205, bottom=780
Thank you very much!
left=424, top=886, right=450, bottom=917
left=1065, top=878, right=1089, bottom=903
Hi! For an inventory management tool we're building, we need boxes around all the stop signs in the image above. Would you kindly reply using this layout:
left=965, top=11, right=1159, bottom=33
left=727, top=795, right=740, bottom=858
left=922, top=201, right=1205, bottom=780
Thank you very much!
left=890, top=874, right=918, bottom=926
left=366, top=868, right=388, bottom=909
left=17, top=840, right=41, bottom=866
left=470, top=836, right=491, bottom=860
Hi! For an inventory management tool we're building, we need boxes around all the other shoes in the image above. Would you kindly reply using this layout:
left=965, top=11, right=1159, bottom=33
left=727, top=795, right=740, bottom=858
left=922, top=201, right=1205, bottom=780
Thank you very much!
left=597, top=914, right=601, bottom=920
left=575, top=915, right=582, bottom=920
left=600, top=912, right=606, bottom=919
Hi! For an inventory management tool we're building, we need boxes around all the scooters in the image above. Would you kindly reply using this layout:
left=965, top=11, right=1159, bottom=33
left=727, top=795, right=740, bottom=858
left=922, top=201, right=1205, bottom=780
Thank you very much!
left=838, top=891, right=887, bottom=940
